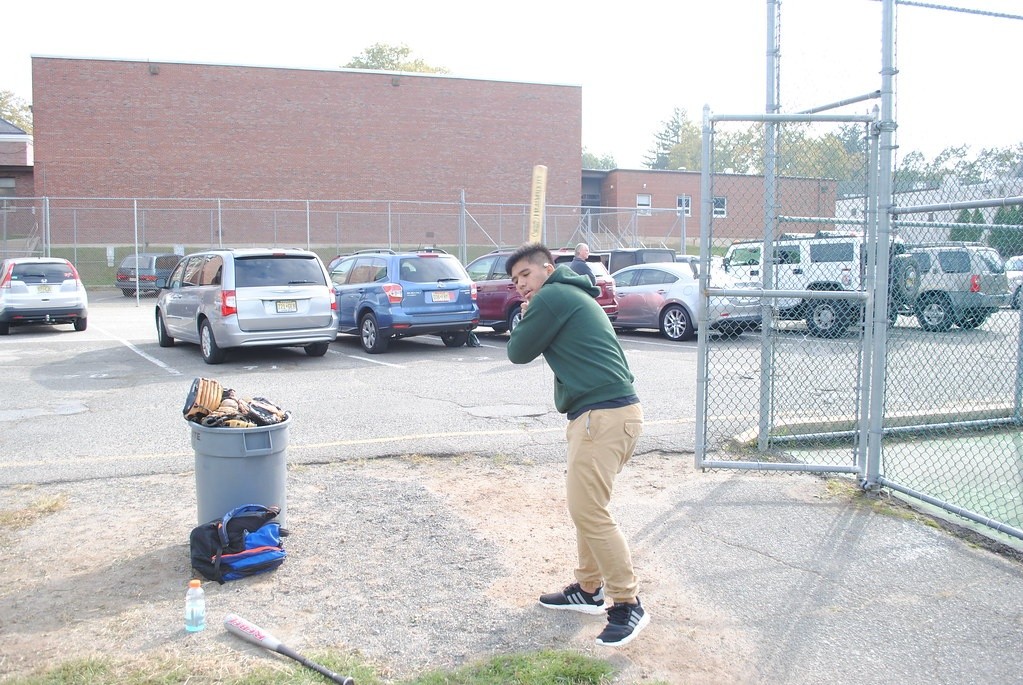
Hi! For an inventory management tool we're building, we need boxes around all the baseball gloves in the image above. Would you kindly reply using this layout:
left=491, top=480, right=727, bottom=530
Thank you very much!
left=182, top=377, right=288, bottom=428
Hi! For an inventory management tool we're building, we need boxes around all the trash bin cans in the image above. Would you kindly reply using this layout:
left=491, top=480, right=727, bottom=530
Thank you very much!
left=186, top=397, right=293, bottom=537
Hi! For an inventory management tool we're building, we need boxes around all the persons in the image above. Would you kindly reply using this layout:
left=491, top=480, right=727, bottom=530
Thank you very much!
left=506, top=243, right=651, bottom=645
left=571, top=243, right=596, bottom=285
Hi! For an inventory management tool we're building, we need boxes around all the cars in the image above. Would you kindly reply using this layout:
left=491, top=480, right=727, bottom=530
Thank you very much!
left=326, top=247, right=480, bottom=354
left=610, top=262, right=766, bottom=340
left=1002, top=255, right=1023, bottom=310
left=465, top=247, right=620, bottom=338
left=154, top=245, right=340, bottom=365
left=116, top=252, right=183, bottom=296
left=0, top=255, right=88, bottom=333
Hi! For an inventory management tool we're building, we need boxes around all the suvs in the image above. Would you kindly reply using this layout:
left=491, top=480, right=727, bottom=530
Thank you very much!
left=715, top=232, right=919, bottom=339
left=592, top=248, right=677, bottom=276
left=906, top=241, right=1013, bottom=332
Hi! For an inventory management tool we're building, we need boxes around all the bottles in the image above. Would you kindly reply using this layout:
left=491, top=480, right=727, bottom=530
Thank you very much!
left=186, top=579, right=207, bottom=631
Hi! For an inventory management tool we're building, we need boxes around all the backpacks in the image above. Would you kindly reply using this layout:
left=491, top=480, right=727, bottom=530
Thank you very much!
left=191, top=503, right=289, bottom=584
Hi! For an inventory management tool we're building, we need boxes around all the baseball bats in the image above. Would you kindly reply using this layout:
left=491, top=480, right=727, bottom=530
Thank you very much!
left=224, top=611, right=355, bottom=685
left=526, top=163, right=549, bottom=306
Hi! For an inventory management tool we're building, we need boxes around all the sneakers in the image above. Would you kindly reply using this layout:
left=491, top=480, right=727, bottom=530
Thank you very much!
left=595, top=596, right=650, bottom=646
left=539, top=580, right=607, bottom=615
